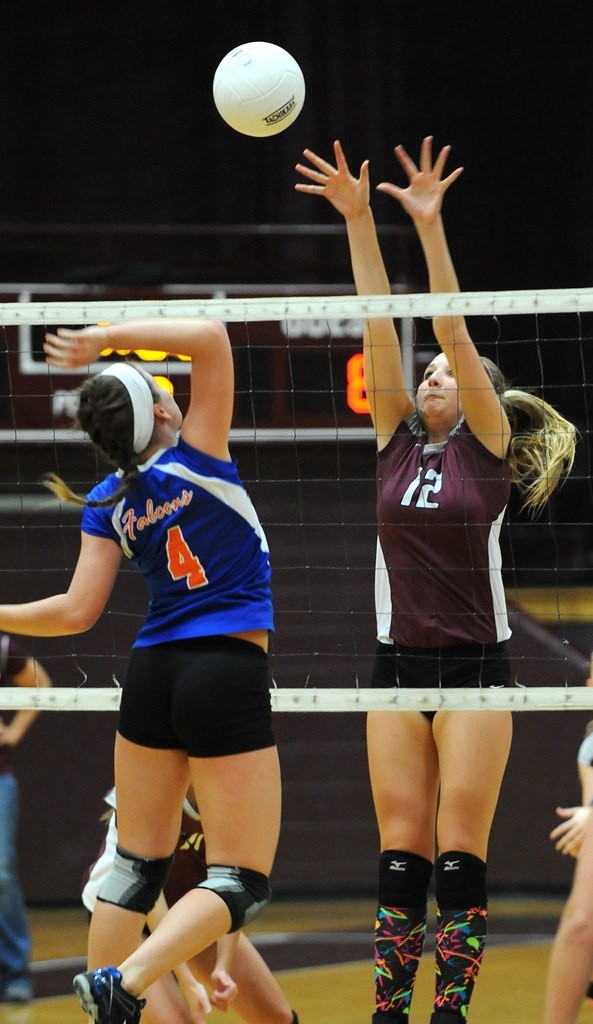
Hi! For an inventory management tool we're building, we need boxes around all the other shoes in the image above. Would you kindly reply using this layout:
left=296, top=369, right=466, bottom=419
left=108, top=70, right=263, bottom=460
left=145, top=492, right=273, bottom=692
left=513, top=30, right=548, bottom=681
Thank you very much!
left=2, top=972, right=33, bottom=1003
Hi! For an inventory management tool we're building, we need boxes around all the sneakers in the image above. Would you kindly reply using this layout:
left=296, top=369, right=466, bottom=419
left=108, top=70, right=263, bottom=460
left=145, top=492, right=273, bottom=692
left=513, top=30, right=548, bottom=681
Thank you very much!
left=73, top=966, right=147, bottom=1024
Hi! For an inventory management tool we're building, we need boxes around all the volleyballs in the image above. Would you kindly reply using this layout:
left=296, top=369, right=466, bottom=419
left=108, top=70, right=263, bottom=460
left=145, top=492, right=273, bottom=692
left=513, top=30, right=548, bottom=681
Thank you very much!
left=212, top=41, right=305, bottom=138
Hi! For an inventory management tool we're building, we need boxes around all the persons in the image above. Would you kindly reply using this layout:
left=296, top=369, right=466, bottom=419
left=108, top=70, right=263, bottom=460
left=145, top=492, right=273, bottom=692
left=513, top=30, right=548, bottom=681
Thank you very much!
left=0, top=632, right=50, bottom=1002
left=0, top=318, right=282, bottom=1024
left=546, top=668, right=593, bottom=1024
left=82, top=781, right=301, bottom=1024
left=296, top=135, right=577, bottom=1024
